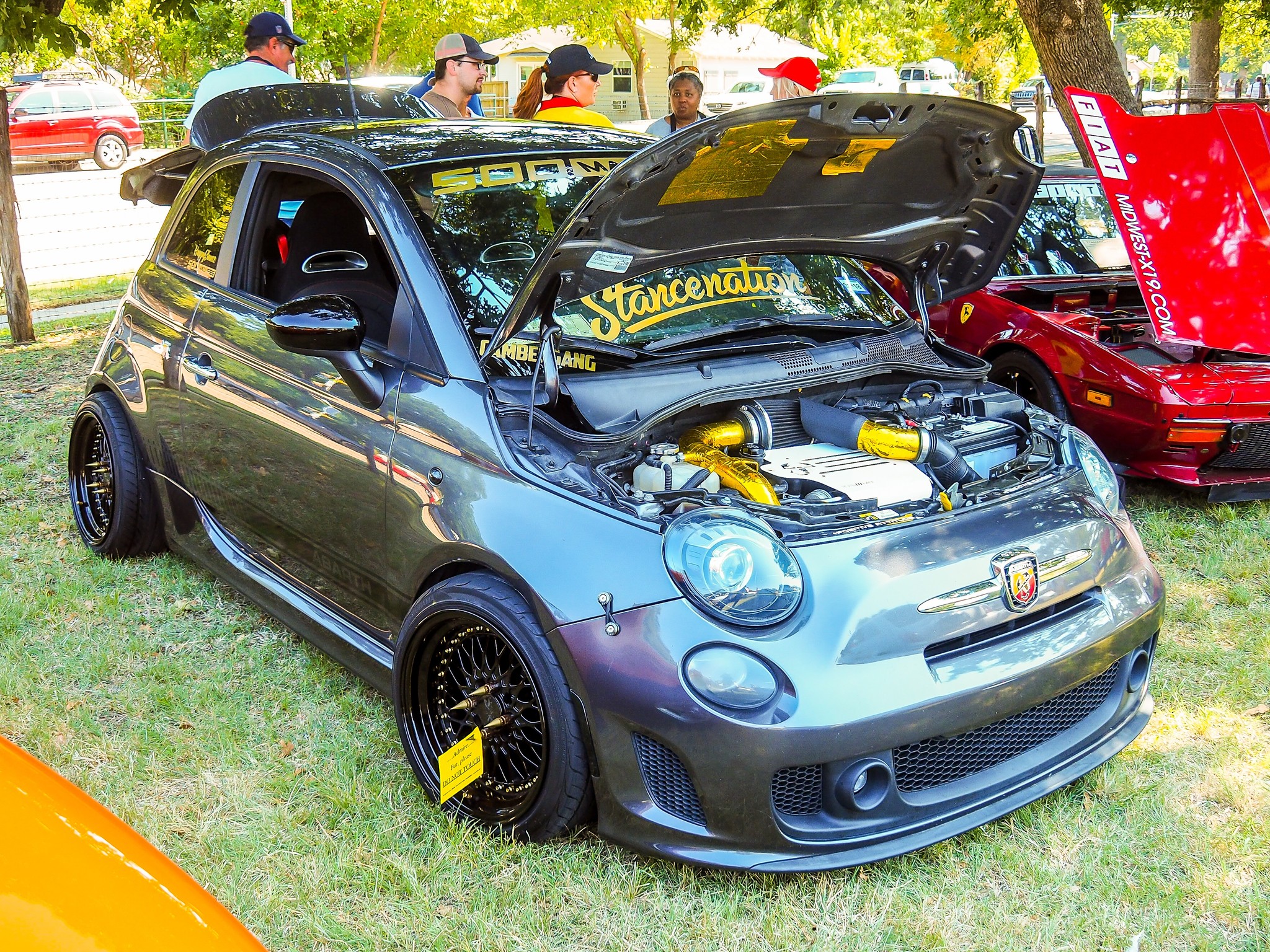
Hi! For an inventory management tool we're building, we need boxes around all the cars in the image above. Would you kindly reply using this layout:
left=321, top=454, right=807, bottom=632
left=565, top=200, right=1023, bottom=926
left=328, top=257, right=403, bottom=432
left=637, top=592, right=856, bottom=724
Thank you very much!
left=65, top=82, right=1163, bottom=874
left=853, top=86, right=1270, bottom=506
left=816, top=68, right=902, bottom=97
left=5, top=72, right=145, bottom=171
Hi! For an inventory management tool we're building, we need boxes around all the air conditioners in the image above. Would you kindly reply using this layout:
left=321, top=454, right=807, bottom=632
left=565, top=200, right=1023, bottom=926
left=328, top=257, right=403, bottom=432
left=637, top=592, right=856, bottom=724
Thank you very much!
left=611, top=100, right=627, bottom=110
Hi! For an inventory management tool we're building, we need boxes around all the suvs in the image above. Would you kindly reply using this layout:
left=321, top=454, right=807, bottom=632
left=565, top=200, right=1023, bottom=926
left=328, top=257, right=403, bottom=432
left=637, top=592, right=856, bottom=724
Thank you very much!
left=1009, top=76, right=1056, bottom=113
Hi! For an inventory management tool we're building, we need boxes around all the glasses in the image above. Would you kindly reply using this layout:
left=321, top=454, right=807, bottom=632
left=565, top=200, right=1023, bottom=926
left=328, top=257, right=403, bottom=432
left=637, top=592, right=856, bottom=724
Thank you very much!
left=672, top=65, right=700, bottom=81
left=457, top=58, right=484, bottom=70
left=277, top=37, right=295, bottom=54
left=576, top=72, right=599, bottom=83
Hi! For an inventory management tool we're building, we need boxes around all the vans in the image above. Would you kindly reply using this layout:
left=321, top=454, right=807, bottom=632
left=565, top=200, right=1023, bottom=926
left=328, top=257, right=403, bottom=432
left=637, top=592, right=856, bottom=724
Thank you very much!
left=899, top=58, right=965, bottom=86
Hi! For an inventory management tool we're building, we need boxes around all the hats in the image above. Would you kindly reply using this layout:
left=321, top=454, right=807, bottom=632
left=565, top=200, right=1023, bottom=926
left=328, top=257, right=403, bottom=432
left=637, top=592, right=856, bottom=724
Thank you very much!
left=545, top=44, right=614, bottom=78
left=434, top=33, right=500, bottom=65
left=244, top=12, right=308, bottom=46
left=758, top=56, right=822, bottom=92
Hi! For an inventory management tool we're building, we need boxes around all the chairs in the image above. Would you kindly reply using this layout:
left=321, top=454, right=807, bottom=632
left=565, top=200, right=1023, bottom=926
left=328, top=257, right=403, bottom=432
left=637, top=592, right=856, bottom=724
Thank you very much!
left=267, top=191, right=397, bottom=347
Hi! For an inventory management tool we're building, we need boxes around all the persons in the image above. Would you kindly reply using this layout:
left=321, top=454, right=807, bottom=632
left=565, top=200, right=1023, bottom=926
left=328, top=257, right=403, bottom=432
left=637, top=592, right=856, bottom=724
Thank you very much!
left=644, top=66, right=713, bottom=139
left=508, top=44, right=617, bottom=128
left=1245, top=75, right=1270, bottom=110
left=757, top=57, right=822, bottom=101
left=404, top=33, right=499, bottom=121
left=180, top=11, right=307, bottom=146
